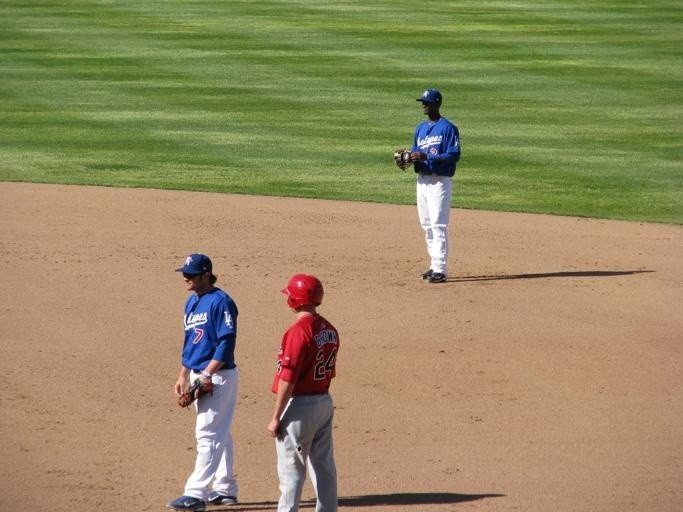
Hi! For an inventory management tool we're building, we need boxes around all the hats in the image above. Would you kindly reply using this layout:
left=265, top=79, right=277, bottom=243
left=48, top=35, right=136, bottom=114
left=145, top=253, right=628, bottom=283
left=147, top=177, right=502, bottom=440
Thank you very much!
left=416, top=90, right=442, bottom=105
left=175, top=254, right=212, bottom=275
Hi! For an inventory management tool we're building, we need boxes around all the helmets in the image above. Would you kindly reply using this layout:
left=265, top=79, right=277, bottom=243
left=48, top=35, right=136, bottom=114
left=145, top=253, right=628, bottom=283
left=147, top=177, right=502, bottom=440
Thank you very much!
left=282, top=274, right=323, bottom=308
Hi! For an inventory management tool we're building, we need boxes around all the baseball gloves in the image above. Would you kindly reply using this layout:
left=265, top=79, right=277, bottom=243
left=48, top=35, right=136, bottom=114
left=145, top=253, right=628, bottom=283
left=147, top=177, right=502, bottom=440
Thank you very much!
left=394, top=149, right=411, bottom=170
left=177, top=378, right=214, bottom=408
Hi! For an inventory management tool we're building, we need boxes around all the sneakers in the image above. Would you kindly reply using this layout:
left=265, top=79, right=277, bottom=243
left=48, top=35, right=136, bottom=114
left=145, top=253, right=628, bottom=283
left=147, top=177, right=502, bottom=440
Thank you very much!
left=207, top=492, right=237, bottom=505
left=168, top=496, right=205, bottom=511
left=420, top=269, right=445, bottom=283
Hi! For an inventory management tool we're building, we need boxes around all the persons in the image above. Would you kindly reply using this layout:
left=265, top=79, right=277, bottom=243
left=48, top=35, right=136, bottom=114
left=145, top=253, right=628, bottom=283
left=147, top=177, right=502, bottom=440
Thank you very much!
left=266, top=273, right=340, bottom=512
left=165, top=253, right=238, bottom=512
left=393, top=89, right=460, bottom=284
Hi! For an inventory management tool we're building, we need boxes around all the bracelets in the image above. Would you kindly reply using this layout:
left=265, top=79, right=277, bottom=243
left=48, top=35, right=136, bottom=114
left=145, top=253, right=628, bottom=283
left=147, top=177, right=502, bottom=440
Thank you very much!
left=201, top=371, right=211, bottom=379
